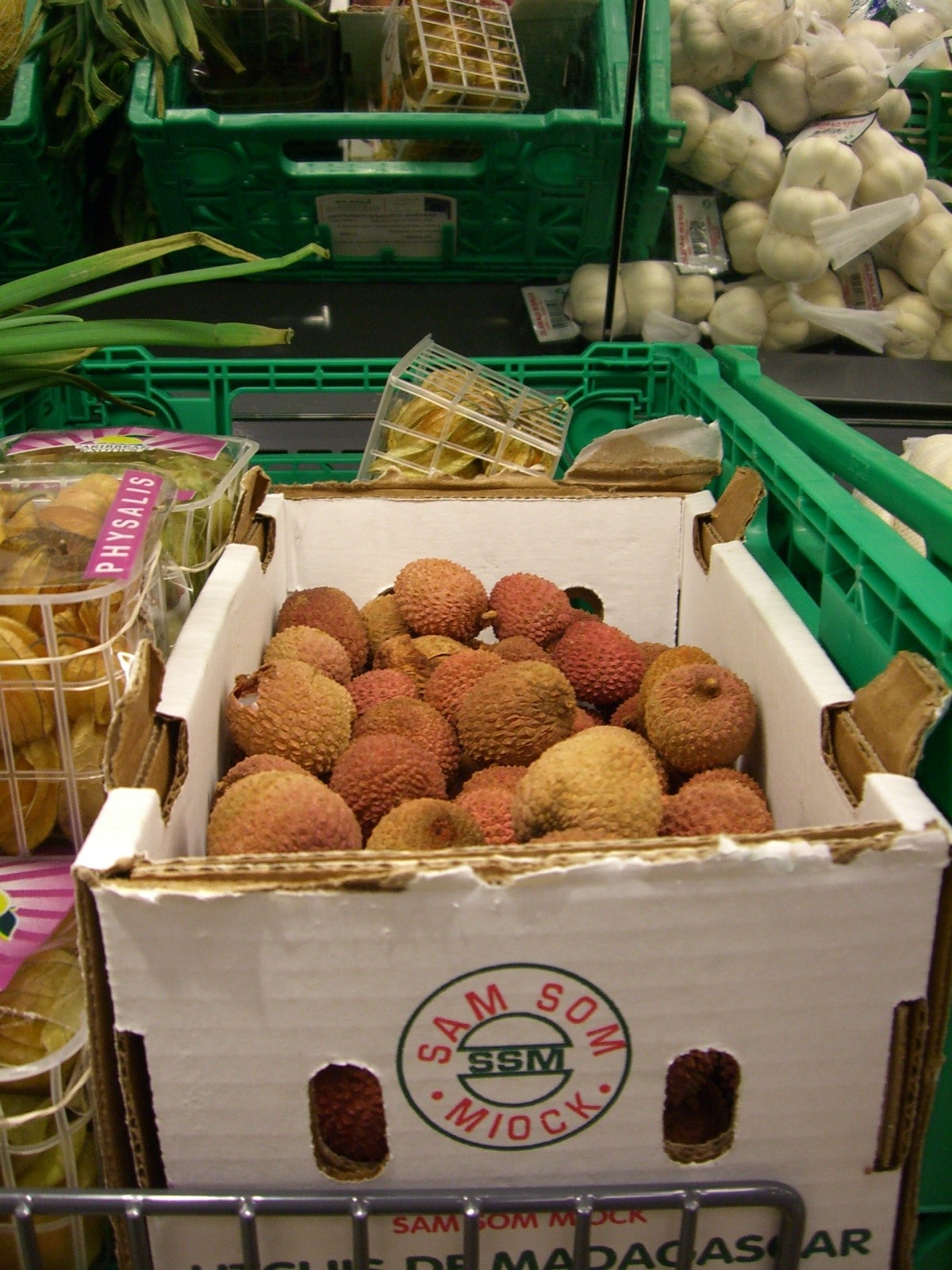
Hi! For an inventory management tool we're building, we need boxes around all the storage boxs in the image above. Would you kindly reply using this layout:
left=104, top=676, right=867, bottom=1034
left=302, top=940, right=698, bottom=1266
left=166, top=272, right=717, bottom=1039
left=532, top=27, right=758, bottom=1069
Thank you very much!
left=0, top=1, right=951, bottom=1268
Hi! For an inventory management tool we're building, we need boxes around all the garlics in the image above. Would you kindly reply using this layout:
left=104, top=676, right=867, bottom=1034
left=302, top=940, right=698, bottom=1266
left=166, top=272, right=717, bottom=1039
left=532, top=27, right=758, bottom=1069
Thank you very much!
left=562, top=0, right=952, bottom=357
left=853, top=434, right=952, bottom=557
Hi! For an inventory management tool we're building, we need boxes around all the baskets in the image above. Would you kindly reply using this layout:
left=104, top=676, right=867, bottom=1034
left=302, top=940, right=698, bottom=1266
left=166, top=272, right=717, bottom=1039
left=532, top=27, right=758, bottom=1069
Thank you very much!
left=0, top=1027, right=90, bottom=1270
left=0, top=534, right=146, bottom=864
left=380, top=0, right=528, bottom=158
left=0, top=429, right=258, bottom=598
left=356, top=333, right=573, bottom=481
